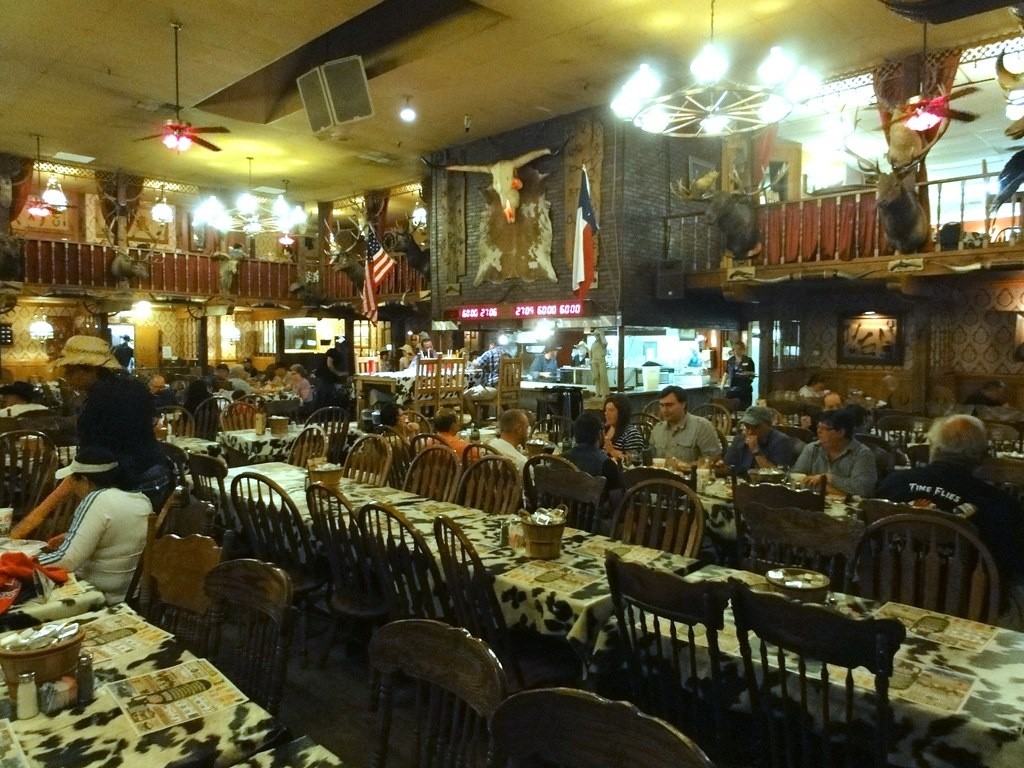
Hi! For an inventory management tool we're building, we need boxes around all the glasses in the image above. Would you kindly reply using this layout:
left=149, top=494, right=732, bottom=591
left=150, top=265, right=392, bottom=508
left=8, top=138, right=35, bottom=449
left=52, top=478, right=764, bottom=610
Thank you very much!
left=816, top=425, right=841, bottom=433
left=291, top=372, right=300, bottom=376
left=411, top=338, right=416, bottom=341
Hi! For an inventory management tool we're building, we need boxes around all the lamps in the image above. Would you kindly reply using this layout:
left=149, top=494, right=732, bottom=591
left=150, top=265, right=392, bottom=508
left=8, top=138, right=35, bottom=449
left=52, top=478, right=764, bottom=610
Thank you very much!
left=610, top=0, right=821, bottom=138
left=901, top=112, right=941, bottom=131
left=163, top=133, right=191, bottom=151
left=28, top=136, right=307, bottom=246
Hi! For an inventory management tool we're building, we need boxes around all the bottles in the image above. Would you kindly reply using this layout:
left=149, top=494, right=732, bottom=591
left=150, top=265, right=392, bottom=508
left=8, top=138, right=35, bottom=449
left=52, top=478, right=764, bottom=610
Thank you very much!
left=76, top=651, right=94, bottom=704
left=829, top=597, right=837, bottom=611
left=0, top=625, right=85, bottom=706
left=499, top=521, right=509, bottom=547
left=16, top=669, right=39, bottom=720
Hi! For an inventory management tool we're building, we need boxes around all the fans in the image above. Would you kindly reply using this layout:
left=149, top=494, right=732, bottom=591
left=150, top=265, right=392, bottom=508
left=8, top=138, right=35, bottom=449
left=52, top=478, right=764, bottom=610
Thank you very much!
left=863, top=22, right=981, bottom=131
left=140, top=22, right=231, bottom=152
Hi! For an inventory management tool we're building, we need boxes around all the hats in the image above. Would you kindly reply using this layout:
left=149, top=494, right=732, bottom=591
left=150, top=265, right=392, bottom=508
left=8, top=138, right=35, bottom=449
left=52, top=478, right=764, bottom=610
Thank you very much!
left=737, top=406, right=773, bottom=425
left=120, top=334, right=133, bottom=341
left=217, top=364, right=230, bottom=371
left=242, top=357, right=251, bottom=362
left=419, top=331, right=430, bottom=345
left=399, top=344, right=414, bottom=355
left=274, top=361, right=285, bottom=369
left=55, top=444, right=117, bottom=480
left=48, top=334, right=124, bottom=370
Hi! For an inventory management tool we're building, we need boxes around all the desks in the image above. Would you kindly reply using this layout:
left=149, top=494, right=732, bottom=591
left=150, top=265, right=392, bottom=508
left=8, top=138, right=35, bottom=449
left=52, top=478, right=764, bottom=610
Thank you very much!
left=157, top=435, right=222, bottom=459
left=594, top=558, right=1024, bottom=768
left=176, top=463, right=421, bottom=568
left=694, top=466, right=860, bottom=567
left=216, top=423, right=359, bottom=466
left=0, top=602, right=286, bottom=768
left=310, top=497, right=702, bottom=685
left=0, top=538, right=106, bottom=631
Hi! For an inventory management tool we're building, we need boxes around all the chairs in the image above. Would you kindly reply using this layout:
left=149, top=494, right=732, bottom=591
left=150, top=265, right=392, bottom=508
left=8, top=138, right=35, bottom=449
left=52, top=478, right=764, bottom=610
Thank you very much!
left=0, top=352, right=1018, bottom=768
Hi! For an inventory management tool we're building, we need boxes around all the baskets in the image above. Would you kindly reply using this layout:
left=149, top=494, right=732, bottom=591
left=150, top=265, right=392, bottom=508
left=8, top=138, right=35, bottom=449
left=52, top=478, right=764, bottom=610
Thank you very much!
left=0, top=623, right=85, bottom=684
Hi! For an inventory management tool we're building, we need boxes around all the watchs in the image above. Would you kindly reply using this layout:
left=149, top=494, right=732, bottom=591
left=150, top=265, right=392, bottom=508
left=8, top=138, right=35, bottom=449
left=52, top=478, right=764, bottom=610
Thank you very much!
left=820, top=473, right=827, bottom=484
left=753, top=449, right=762, bottom=456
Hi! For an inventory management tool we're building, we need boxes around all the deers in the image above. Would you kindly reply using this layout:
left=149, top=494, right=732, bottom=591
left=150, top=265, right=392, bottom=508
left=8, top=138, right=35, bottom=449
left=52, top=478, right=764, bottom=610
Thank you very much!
left=0, top=228, right=31, bottom=284
left=208, top=248, right=249, bottom=290
left=415, top=135, right=575, bottom=224
left=306, top=193, right=394, bottom=297
left=667, top=159, right=791, bottom=257
left=841, top=74, right=951, bottom=251
left=95, top=186, right=166, bottom=279
left=381, top=209, right=428, bottom=282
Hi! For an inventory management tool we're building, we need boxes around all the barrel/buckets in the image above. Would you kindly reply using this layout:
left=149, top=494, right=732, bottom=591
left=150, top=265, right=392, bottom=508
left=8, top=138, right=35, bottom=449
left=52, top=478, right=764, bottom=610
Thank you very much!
left=765, top=568, right=830, bottom=603
left=269, top=419, right=289, bottom=436
left=307, top=465, right=343, bottom=496
left=522, top=515, right=567, bottom=560
left=748, top=468, right=786, bottom=485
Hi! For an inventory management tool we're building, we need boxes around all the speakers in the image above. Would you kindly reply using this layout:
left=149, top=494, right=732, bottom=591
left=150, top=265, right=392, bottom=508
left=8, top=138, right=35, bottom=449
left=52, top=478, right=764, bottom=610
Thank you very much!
left=296, top=53, right=375, bottom=134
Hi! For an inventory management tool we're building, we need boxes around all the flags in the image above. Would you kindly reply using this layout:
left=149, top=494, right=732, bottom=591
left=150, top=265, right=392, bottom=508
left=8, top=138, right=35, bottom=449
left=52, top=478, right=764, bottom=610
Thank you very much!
left=362, top=225, right=397, bottom=326
left=572, top=168, right=601, bottom=312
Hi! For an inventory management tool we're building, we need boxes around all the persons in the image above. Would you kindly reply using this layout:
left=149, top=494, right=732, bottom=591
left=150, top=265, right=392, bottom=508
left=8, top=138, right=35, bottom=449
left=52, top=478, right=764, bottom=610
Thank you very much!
left=0, top=380, right=50, bottom=446
left=149, top=374, right=182, bottom=408
left=263, top=363, right=314, bottom=420
left=589, top=328, right=610, bottom=399
left=385, top=386, right=1024, bottom=621
left=111, top=335, right=133, bottom=368
left=241, top=357, right=257, bottom=377
left=314, top=337, right=348, bottom=422
left=9, top=336, right=174, bottom=552
left=283, top=247, right=295, bottom=263
left=379, top=340, right=437, bottom=372
left=231, top=242, right=248, bottom=258
left=464, top=336, right=589, bottom=426
left=720, top=341, right=755, bottom=410
left=28, top=443, right=153, bottom=605
left=178, top=363, right=262, bottom=442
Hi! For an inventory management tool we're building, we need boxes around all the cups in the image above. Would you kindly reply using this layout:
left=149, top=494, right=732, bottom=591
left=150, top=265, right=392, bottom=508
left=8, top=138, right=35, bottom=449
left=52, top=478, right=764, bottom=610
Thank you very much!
left=0, top=508, right=14, bottom=536
left=632, top=453, right=642, bottom=468
left=642, top=366, right=660, bottom=388
left=652, top=458, right=666, bottom=469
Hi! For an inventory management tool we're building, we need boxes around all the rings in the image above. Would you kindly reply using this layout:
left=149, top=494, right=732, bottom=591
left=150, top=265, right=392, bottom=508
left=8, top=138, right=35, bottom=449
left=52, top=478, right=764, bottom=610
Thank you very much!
left=747, top=438, right=749, bottom=440
